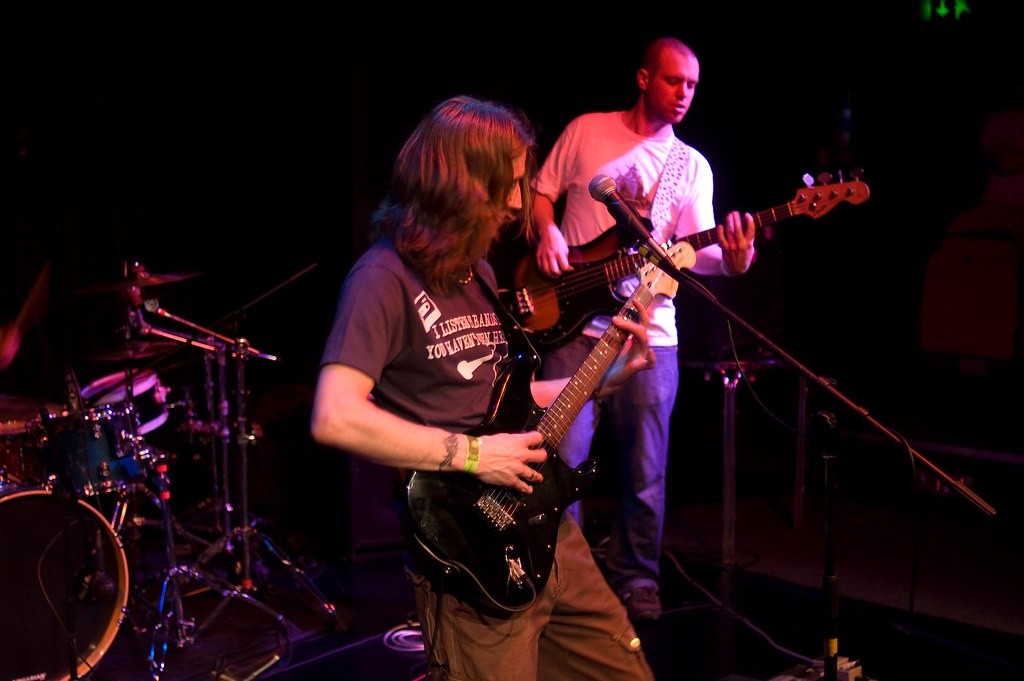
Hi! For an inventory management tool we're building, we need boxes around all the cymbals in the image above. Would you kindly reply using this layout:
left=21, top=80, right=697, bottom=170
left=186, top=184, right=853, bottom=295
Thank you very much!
left=90, top=340, right=179, bottom=360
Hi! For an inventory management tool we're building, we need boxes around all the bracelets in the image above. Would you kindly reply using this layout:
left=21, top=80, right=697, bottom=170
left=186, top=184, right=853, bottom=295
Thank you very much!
left=463, top=435, right=480, bottom=474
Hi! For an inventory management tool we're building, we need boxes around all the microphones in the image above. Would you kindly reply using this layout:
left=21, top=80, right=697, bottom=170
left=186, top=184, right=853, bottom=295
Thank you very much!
left=588, top=174, right=677, bottom=271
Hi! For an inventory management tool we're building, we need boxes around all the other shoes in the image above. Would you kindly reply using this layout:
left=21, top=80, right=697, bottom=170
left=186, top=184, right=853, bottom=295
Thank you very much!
left=618, top=576, right=662, bottom=620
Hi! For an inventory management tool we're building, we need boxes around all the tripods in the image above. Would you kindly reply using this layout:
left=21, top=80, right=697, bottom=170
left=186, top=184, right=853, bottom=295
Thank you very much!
left=130, top=342, right=349, bottom=681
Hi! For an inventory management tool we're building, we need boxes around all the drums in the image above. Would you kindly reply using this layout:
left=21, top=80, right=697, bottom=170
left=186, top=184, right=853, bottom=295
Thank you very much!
left=0, top=400, right=78, bottom=488
left=1, top=487, right=130, bottom=681
left=81, top=370, right=169, bottom=436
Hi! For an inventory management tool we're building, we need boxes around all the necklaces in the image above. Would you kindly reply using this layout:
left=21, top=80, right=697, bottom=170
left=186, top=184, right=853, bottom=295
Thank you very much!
left=457, top=264, right=473, bottom=284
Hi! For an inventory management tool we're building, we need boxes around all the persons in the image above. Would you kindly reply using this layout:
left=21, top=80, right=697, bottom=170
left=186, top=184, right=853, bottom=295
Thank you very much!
left=310, top=96, right=657, bottom=681
left=527, top=37, right=756, bottom=619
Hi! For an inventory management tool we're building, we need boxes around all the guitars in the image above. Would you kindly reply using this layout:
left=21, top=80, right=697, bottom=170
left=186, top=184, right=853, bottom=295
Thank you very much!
left=403, top=239, right=696, bottom=622
left=506, top=165, right=870, bottom=352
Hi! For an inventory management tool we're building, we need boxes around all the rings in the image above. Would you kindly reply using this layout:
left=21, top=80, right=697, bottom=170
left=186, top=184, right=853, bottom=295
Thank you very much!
left=527, top=466, right=534, bottom=480
